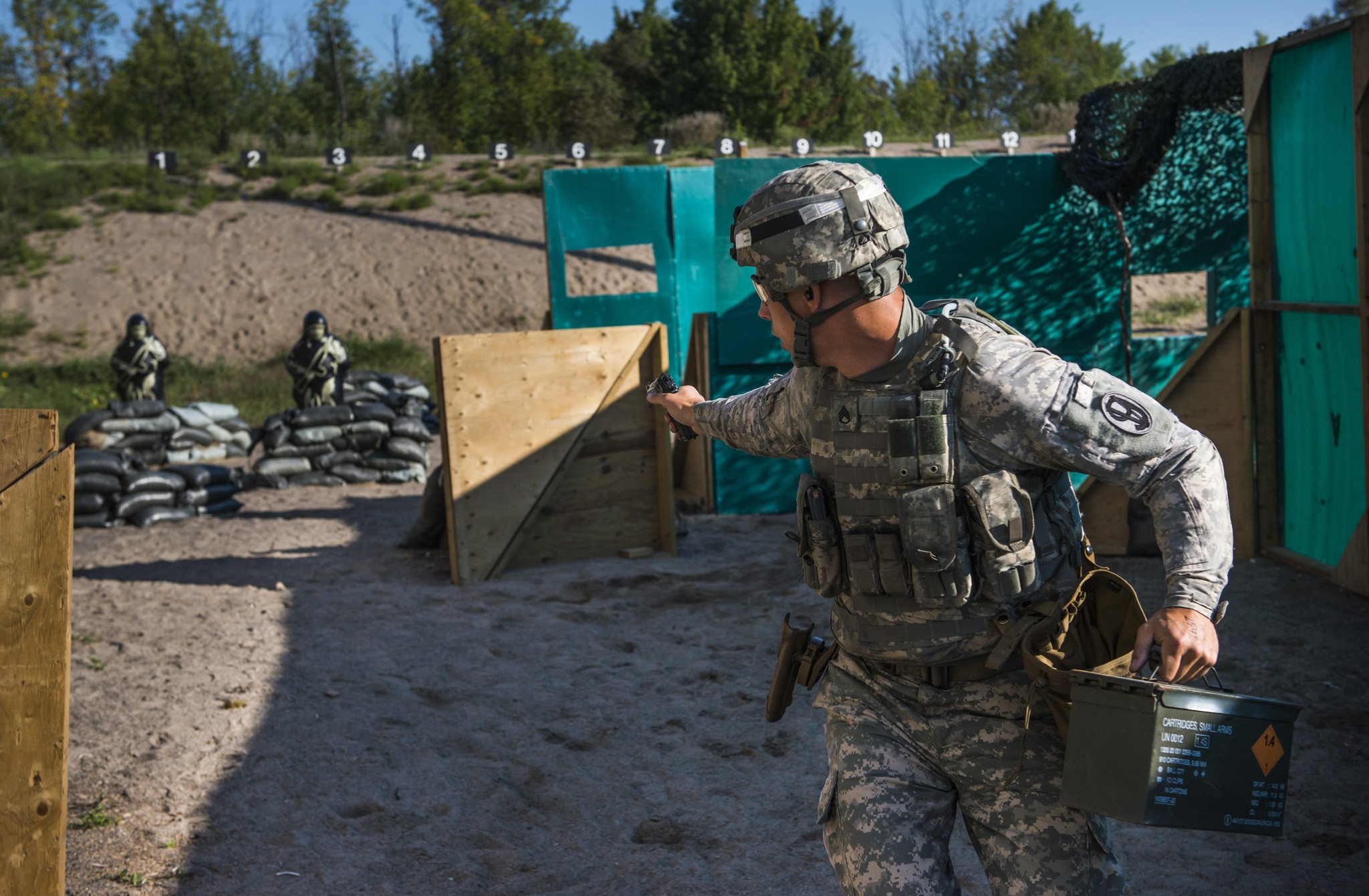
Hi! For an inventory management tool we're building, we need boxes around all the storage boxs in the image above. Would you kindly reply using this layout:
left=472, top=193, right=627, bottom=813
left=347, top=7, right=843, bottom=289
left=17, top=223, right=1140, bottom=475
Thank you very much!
left=1058, top=660, right=1304, bottom=837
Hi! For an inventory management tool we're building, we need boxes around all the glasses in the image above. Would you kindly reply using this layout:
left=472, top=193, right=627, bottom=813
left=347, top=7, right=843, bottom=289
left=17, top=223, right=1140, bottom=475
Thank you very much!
left=750, top=273, right=775, bottom=306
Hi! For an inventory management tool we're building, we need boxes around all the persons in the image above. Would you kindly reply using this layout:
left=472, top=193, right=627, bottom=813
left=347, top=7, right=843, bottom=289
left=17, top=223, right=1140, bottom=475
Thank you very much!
left=285, top=308, right=347, bottom=410
left=112, top=312, right=170, bottom=405
left=647, top=163, right=1235, bottom=896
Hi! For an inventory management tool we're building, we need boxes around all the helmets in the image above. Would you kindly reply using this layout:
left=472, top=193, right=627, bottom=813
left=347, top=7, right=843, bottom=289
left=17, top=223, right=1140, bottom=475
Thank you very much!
left=127, top=314, right=147, bottom=327
left=304, top=310, right=327, bottom=325
left=730, top=159, right=911, bottom=300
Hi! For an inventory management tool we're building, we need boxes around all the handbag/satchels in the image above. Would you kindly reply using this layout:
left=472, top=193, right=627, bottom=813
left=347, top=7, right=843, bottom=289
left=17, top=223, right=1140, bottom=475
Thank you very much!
left=1022, top=566, right=1148, bottom=740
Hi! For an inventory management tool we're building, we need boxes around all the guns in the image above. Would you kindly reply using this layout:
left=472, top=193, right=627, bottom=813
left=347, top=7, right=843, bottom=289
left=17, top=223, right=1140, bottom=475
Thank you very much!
left=309, top=349, right=383, bottom=402
left=645, top=372, right=698, bottom=442
